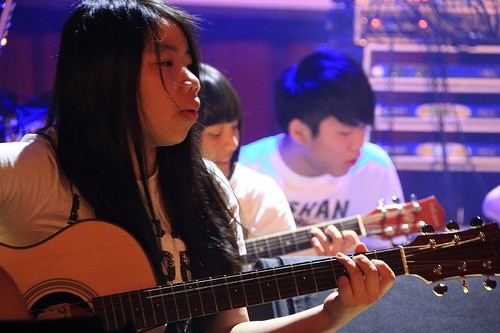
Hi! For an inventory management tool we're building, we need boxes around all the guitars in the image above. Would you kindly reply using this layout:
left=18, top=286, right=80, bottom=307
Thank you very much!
left=241, top=193, right=447, bottom=265
left=0, top=215, right=500, bottom=333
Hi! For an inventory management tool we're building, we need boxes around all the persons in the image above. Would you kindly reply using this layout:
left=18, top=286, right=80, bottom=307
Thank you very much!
left=0, top=0, right=397, bottom=333
left=192, top=62, right=368, bottom=275
left=227, top=50, right=411, bottom=272
